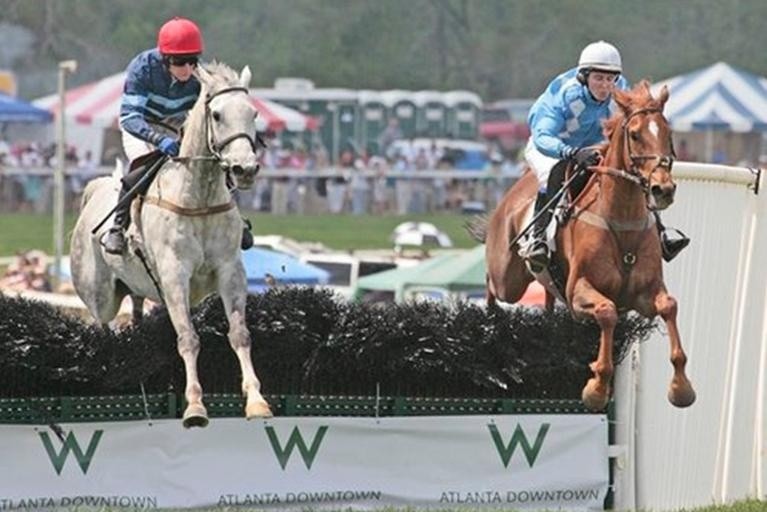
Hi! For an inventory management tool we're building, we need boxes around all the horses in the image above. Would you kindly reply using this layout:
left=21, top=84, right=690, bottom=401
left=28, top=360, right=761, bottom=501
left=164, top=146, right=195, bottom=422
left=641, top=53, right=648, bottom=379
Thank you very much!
left=462, top=78, right=697, bottom=410
left=69, top=63, right=273, bottom=428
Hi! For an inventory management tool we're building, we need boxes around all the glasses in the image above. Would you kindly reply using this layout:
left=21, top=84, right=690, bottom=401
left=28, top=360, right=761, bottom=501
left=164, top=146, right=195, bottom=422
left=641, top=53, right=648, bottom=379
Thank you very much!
left=165, top=53, right=197, bottom=66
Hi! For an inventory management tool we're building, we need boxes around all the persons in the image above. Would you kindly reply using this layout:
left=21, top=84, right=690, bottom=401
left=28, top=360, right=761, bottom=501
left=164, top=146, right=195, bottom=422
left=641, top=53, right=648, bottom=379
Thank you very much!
left=711, top=143, right=729, bottom=161
left=105, top=17, right=253, bottom=256
left=0, top=143, right=94, bottom=209
left=382, top=118, right=405, bottom=148
left=525, top=42, right=692, bottom=273
left=676, top=136, right=701, bottom=162
left=357, top=140, right=515, bottom=212
left=255, top=139, right=355, bottom=216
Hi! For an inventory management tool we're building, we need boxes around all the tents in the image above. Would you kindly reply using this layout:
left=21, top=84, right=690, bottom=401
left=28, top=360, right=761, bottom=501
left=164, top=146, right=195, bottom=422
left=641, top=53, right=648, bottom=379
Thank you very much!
left=37, top=72, right=318, bottom=135
left=243, top=247, right=330, bottom=292
left=1, top=93, right=53, bottom=127
left=651, top=58, right=766, bottom=160
left=396, top=240, right=490, bottom=294
left=358, top=251, right=457, bottom=293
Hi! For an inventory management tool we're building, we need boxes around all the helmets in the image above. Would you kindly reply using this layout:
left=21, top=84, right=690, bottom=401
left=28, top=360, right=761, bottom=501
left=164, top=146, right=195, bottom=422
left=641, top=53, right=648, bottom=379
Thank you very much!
left=577, top=42, right=622, bottom=73
left=158, top=19, right=203, bottom=55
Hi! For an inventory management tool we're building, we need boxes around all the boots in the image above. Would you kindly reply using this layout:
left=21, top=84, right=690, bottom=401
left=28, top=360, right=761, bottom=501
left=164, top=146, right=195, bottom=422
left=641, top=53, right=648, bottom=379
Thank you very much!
left=658, top=226, right=691, bottom=261
left=107, top=186, right=131, bottom=254
left=528, top=192, right=549, bottom=273
